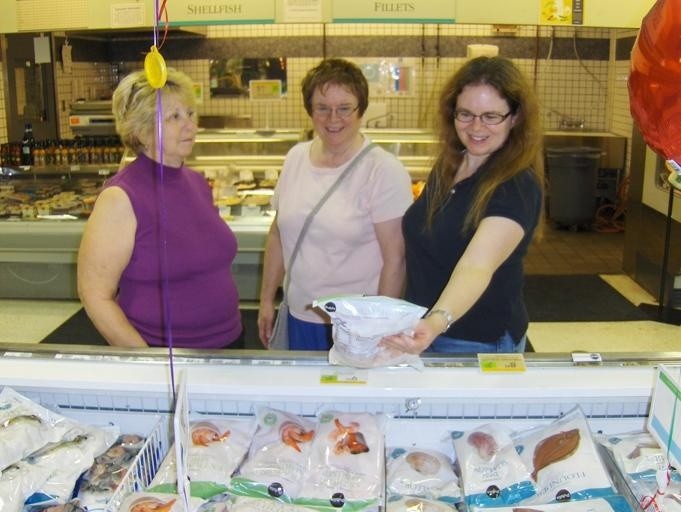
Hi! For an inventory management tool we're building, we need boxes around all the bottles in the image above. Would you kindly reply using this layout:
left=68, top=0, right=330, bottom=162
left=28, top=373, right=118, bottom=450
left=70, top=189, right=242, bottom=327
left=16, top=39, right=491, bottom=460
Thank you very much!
left=0, top=123, right=126, bottom=163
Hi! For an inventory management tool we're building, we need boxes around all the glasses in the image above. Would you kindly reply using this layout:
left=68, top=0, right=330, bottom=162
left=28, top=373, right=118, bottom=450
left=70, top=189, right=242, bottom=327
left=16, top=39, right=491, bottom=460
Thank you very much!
left=314, top=103, right=359, bottom=115
left=456, top=110, right=516, bottom=126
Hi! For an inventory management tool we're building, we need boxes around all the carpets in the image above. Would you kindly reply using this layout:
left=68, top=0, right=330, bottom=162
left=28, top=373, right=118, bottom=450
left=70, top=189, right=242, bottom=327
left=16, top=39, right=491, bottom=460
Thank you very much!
left=40, top=273, right=650, bottom=353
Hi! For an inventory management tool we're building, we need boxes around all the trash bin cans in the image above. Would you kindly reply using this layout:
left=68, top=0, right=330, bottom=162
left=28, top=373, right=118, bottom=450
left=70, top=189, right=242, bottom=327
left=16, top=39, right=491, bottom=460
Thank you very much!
left=546, top=145, right=608, bottom=233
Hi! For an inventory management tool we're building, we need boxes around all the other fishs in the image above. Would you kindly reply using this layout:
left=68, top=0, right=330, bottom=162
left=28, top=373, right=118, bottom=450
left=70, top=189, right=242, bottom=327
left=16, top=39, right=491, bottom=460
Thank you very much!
left=530, top=427, right=581, bottom=483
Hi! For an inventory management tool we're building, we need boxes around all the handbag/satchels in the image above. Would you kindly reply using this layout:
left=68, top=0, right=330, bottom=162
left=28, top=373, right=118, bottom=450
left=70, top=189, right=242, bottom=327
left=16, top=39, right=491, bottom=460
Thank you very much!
left=266, top=301, right=291, bottom=351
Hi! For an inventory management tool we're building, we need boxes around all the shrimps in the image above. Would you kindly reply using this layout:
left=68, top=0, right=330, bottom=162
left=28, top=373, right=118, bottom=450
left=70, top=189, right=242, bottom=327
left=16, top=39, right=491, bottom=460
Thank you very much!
left=191, top=427, right=230, bottom=448
left=278, top=422, right=315, bottom=452
left=328, top=418, right=360, bottom=442
left=132, top=498, right=178, bottom=512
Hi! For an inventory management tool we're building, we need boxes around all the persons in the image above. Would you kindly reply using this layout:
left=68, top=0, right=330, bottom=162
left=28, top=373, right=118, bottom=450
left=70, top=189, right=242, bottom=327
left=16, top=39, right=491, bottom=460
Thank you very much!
left=76, top=66, right=246, bottom=348
left=257, top=59, right=412, bottom=350
left=378, top=56, right=543, bottom=356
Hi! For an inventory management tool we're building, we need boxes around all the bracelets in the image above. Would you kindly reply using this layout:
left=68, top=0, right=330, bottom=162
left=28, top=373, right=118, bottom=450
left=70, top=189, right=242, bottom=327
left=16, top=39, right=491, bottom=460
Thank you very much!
left=427, top=307, right=455, bottom=333
